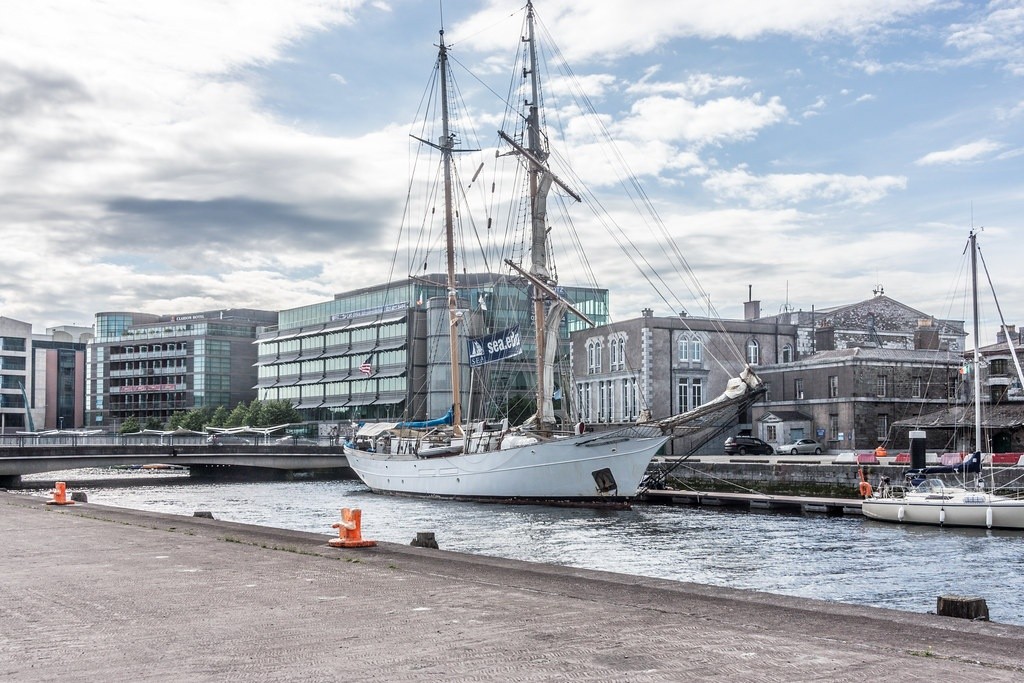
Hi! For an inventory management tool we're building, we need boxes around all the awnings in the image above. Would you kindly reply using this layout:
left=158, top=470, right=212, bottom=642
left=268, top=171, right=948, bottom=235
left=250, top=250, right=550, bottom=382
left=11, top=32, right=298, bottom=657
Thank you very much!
left=891, top=405, right=1024, bottom=429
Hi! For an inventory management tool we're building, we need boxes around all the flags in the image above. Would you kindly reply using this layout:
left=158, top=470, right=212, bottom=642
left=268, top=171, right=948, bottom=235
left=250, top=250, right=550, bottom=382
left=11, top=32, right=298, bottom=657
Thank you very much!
left=960, top=364, right=971, bottom=375
left=359, top=353, right=372, bottom=376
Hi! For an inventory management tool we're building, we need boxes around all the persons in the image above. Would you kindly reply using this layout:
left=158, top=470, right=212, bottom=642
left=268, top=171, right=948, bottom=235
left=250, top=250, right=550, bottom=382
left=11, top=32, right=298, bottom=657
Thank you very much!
left=330, top=426, right=339, bottom=445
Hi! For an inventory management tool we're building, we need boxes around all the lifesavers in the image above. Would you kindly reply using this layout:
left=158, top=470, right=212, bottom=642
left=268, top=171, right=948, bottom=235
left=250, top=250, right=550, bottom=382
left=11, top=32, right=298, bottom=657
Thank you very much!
left=859, top=482, right=871, bottom=496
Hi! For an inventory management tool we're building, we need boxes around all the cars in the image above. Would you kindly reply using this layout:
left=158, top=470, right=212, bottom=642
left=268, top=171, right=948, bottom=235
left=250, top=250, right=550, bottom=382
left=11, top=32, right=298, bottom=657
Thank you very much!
left=276, top=436, right=318, bottom=446
left=777, top=438, right=824, bottom=455
left=207, top=433, right=250, bottom=446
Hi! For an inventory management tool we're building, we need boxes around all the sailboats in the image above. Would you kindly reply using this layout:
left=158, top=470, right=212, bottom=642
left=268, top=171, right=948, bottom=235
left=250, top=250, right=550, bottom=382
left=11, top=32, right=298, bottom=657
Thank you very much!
left=865, top=228, right=1024, bottom=528
left=341, top=0, right=769, bottom=512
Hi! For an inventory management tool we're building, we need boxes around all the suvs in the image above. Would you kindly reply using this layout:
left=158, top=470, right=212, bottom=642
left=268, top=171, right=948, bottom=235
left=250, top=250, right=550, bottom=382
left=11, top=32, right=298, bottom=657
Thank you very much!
left=724, top=435, right=773, bottom=456
